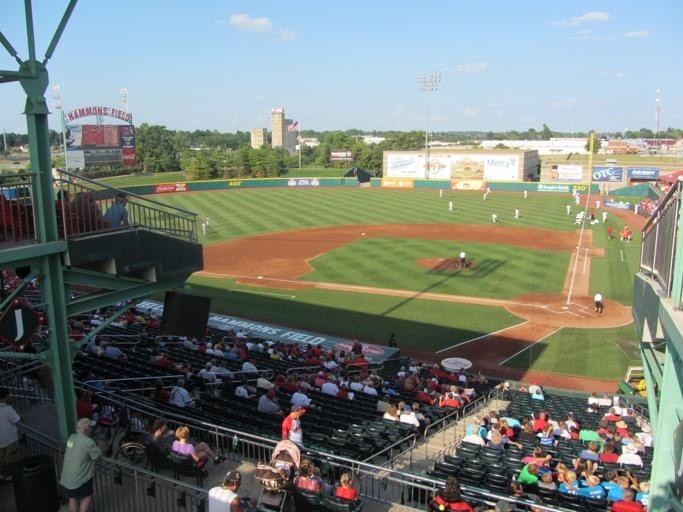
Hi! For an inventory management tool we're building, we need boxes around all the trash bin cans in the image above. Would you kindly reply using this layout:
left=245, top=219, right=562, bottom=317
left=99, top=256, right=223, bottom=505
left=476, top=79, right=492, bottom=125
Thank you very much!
left=12, top=455, right=60, bottom=512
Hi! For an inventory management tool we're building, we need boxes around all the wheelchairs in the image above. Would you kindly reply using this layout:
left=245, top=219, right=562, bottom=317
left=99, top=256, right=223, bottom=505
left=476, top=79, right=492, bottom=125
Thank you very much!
left=115, top=428, right=154, bottom=470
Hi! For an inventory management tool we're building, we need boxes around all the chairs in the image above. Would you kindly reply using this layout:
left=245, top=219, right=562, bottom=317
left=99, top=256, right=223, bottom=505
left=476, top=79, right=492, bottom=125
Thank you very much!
left=78, top=351, right=655, bottom=512
left=0, top=192, right=83, bottom=246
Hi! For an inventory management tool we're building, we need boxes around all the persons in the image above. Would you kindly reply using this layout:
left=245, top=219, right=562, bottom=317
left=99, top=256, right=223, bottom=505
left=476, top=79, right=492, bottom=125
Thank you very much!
left=1, top=270, right=490, bottom=510
left=54, top=189, right=130, bottom=239
left=440, top=188, right=443, bottom=198
left=594, top=291, right=603, bottom=313
left=492, top=213, right=497, bottom=223
left=448, top=200, right=453, bottom=211
left=455, top=250, right=466, bottom=269
left=464, top=382, right=650, bottom=511
left=524, top=190, right=527, bottom=199
left=201, top=215, right=209, bottom=235
left=565, top=181, right=674, bottom=243
left=483, top=187, right=492, bottom=201
left=515, top=208, right=520, bottom=221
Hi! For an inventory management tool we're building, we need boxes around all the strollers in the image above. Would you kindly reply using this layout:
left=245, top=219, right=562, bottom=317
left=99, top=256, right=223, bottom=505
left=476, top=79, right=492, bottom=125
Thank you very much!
left=252, top=440, right=300, bottom=512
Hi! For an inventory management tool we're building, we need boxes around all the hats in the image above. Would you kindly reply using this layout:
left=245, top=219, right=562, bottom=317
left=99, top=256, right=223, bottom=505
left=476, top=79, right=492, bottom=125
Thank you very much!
left=153, top=419, right=168, bottom=429
left=566, top=469, right=577, bottom=482
left=226, top=470, right=241, bottom=482
left=76, top=418, right=97, bottom=428
left=292, top=404, right=306, bottom=414
left=589, top=474, right=600, bottom=485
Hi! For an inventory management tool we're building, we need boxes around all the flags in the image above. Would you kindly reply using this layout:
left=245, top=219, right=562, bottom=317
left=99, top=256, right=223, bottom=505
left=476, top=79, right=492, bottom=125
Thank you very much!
left=52, top=85, right=61, bottom=110
left=120, top=88, right=128, bottom=112
left=287, top=120, right=299, bottom=132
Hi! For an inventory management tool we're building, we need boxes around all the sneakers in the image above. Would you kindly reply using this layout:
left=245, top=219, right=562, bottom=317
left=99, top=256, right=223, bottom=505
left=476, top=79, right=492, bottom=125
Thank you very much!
left=214, top=456, right=227, bottom=466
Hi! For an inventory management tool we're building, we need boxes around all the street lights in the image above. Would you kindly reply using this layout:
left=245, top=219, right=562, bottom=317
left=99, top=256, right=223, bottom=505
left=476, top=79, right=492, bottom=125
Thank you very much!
left=416, top=70, right=441, bottom=180
